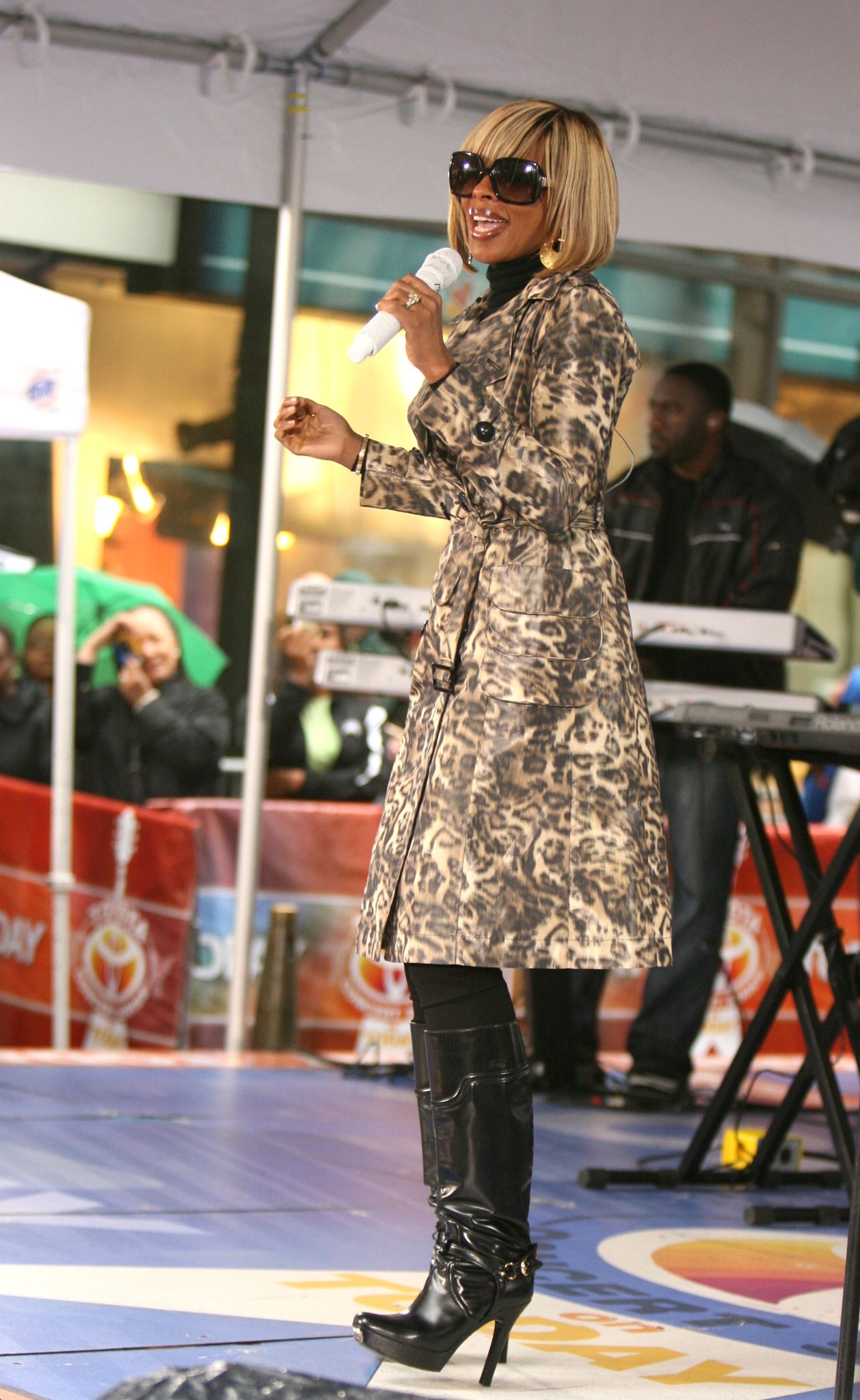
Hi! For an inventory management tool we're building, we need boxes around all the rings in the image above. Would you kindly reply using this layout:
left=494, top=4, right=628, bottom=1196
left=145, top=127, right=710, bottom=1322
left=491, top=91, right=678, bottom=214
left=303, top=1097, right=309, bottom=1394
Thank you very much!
left=405, top=290, right=420, bottom=309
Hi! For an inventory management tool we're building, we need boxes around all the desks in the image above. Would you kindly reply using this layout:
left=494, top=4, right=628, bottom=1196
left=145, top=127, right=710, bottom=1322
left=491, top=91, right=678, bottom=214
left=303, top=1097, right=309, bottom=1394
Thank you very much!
left=580, top=719, right=860, bottom=1226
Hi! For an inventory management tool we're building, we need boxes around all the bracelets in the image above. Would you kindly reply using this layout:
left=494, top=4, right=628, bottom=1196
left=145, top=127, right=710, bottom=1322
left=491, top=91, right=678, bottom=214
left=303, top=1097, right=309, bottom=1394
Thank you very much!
left=356, top=433, right=369, bottom=476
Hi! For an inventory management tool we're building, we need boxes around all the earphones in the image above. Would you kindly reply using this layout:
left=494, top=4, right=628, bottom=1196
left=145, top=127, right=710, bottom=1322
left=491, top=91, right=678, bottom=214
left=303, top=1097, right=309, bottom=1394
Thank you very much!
left=708, top=417, right=715, bottom=427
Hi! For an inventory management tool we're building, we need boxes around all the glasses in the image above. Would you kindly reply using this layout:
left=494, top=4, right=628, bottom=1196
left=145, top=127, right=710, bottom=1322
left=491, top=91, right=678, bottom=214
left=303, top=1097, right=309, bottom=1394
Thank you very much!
left=449, top=150, right=550, bottom=206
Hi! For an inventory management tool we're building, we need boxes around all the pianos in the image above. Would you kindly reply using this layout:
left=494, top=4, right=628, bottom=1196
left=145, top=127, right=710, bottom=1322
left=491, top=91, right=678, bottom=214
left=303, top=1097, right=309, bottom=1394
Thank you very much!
left=290, top=581, right=856, bottom=1229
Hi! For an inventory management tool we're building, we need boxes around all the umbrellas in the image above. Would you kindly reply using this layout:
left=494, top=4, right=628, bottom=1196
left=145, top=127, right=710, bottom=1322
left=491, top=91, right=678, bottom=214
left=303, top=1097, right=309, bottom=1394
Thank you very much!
left=0, top=566, right=227, bottom=691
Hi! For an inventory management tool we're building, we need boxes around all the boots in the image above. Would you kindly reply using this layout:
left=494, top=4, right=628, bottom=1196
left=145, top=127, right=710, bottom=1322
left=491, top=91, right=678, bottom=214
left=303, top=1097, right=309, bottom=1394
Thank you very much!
left=351, top=1020, right=543, bottom=1387
left=410, top=1020, right=510, bottom=1364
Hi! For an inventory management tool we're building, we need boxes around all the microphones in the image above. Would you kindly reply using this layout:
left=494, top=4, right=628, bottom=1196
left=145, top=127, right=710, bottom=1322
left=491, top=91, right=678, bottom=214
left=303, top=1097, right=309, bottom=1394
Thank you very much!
left=349, top=247, right=464, bottom=362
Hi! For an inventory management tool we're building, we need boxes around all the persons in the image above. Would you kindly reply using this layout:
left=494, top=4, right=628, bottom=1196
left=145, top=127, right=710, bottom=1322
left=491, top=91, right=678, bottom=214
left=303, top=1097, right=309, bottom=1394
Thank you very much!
left=528, top=361, right=800, bottom=1115
left=0, top=605, right=420, bottom=810
left=275, top=100, right=635, bottom=1388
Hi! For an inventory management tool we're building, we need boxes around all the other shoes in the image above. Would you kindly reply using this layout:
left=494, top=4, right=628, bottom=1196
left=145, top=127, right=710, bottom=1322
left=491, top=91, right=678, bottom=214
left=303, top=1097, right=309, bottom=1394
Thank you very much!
left=596, top=1072, right=695, bottom=1113
left=529, top=1056, right=604, bottom=1090
